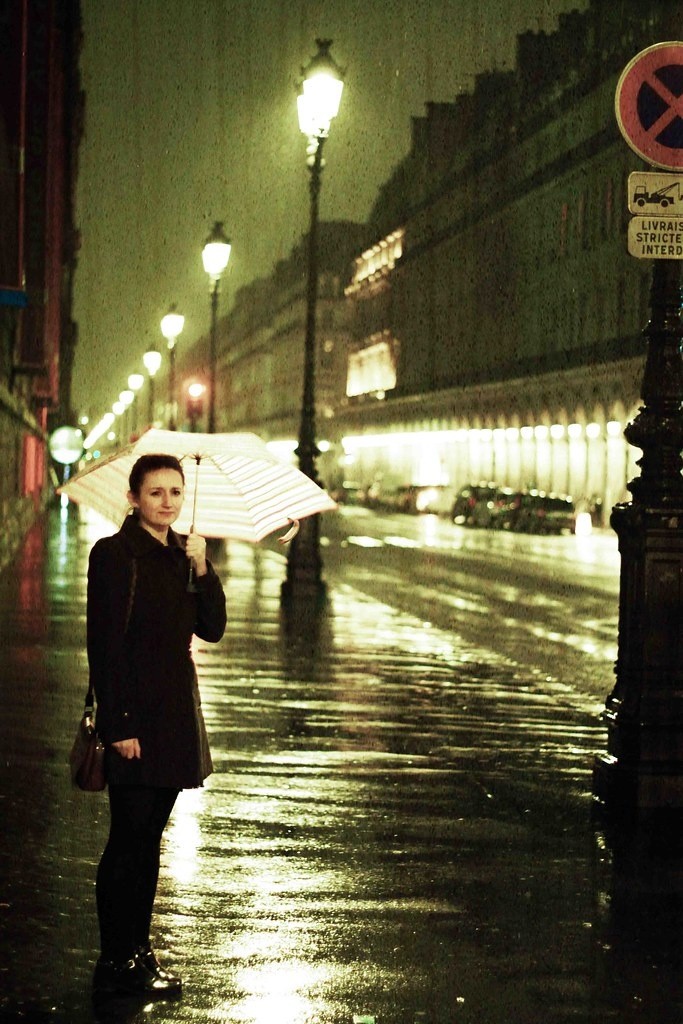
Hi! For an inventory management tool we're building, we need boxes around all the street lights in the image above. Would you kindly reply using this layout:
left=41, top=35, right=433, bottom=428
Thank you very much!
left=159, top=303, right=188, bottom=430
left=198, top=218, right=237, bottom=432
left=277, top=32, right=348, bottom=605
left=85, top=343, right=165, bottom=463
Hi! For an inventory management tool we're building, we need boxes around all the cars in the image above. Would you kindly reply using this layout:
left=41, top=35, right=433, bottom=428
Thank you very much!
left=326, top=478, right=577, bottom=536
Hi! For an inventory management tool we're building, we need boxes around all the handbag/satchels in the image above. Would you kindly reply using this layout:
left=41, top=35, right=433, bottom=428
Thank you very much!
left=70, top=716, right=107, bottom=793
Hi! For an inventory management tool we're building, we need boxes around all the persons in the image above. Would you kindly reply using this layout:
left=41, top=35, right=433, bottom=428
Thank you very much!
left=69, top=453, right=228, bottom=996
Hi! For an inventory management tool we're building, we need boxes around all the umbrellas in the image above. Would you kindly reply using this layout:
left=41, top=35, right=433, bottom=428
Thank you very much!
left=54, top=427, right=338, bottom=593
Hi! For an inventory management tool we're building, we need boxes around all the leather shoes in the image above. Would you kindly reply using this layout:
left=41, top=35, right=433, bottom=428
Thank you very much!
left=93, top=946, right=182, bottom=994
